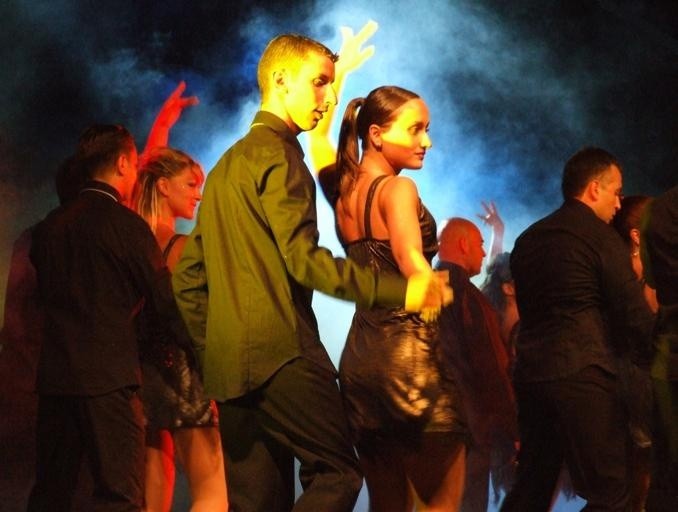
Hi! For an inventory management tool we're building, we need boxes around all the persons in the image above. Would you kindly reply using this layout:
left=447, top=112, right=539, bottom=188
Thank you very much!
left=26, top=122, right=195, bottom=512
left=129, top=81, right=228, bottom=512
left=0, top=158, right=94, bottom=512
left=309, top=20, right=471, bottom=512
left=432, top=218, right=519, bottom=512
left=479, top=147, right=678, bottom=511
left=170, top=32, right=450, bottom=512
